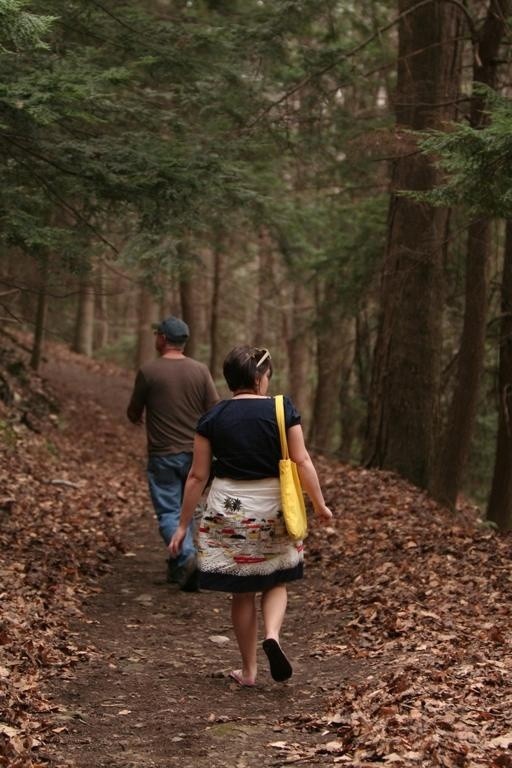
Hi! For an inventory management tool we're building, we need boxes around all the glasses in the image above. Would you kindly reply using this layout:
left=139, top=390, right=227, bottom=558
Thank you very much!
left=256, top=346, right=272, bottom=367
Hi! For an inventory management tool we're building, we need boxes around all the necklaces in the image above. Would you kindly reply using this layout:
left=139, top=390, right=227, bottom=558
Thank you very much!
left=232, top=391, right=258, bottom=396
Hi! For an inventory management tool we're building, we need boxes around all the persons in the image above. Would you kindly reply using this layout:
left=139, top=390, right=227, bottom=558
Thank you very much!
left=126, top=314, right=222, bottom=594
left=167, top=344, right=338, bottom=686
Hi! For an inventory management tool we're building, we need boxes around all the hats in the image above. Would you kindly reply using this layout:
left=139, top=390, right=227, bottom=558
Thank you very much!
left=151, top=318, right=190, bottom=342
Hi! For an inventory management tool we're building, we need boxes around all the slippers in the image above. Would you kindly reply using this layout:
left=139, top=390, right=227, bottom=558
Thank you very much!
left=230, top=639, right=293, bottom=688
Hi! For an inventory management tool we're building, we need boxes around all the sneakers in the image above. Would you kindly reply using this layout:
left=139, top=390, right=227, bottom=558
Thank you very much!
left=166, top=556, right=199, bottom=592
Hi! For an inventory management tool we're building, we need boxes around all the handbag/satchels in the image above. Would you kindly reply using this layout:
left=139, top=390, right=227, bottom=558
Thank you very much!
left=279, top=459, right=307, bottom=541
